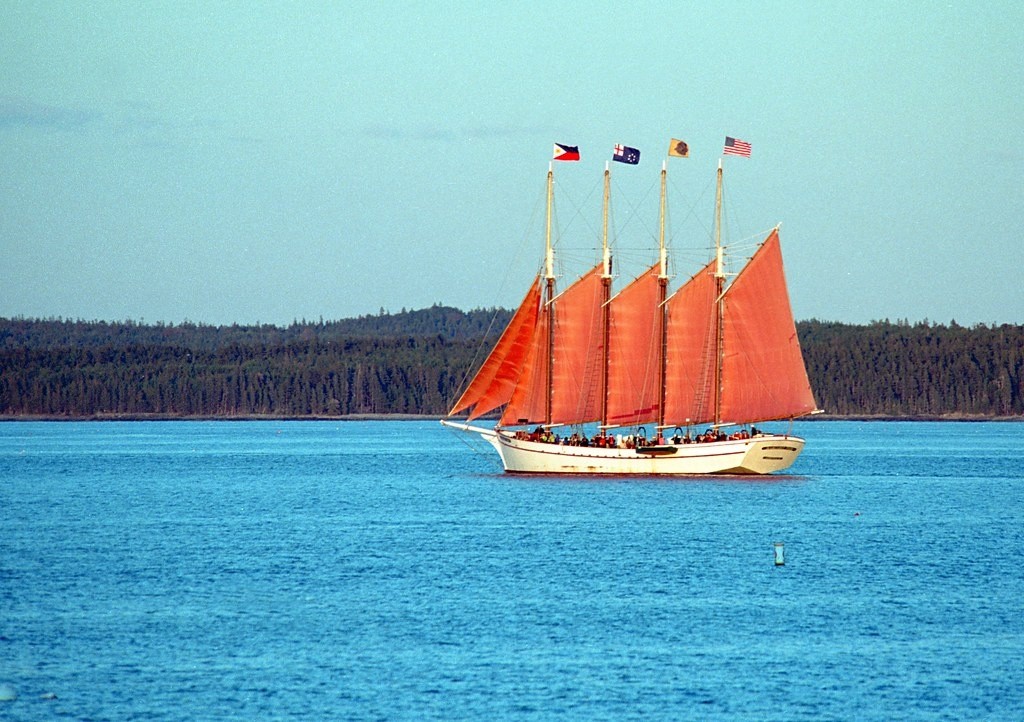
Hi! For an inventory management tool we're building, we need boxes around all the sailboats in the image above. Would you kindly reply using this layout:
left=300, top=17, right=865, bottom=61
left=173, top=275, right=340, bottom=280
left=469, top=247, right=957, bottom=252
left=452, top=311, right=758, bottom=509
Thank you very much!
left=440, top=137, right=826, bottom=480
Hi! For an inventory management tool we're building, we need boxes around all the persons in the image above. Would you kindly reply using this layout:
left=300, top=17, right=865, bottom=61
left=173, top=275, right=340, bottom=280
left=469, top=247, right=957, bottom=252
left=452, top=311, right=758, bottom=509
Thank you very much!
left=530, top=425, right=762, bottom=450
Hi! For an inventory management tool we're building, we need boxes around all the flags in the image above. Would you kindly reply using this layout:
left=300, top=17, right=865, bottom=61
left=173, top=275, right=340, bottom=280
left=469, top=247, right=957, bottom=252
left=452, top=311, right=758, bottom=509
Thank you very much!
left=612, top=143, right=641, bottom=165
left=553, top=143, right=581, bottom=161
left=723, top=135, right=752, bottom=158
left=667, top=138, right=689, bottom=158
